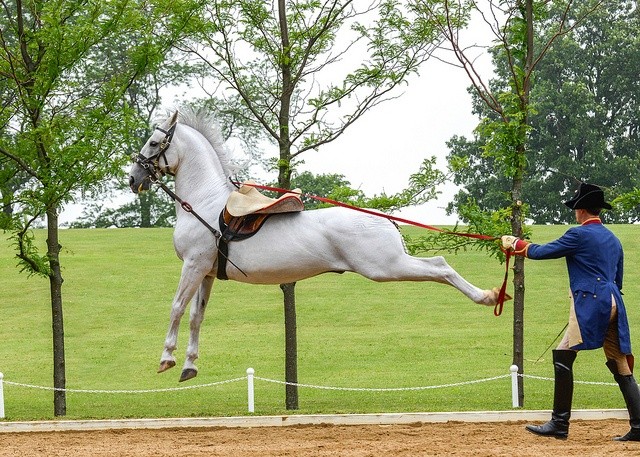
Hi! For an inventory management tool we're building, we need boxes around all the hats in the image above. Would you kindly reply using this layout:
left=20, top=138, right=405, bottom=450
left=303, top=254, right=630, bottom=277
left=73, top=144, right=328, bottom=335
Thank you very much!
left=564, top=182, right=613, bottom=210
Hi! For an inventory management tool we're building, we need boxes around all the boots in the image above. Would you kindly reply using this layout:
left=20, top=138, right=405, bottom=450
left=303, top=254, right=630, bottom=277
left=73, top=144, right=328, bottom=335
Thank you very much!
left=525, top=350, right=577, bottom=440
left=605, top=358, right=640, bottom=441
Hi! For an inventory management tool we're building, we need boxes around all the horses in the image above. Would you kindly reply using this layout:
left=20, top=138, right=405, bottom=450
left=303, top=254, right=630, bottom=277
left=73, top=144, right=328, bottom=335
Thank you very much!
left=128, top=107, right=512, bottom=382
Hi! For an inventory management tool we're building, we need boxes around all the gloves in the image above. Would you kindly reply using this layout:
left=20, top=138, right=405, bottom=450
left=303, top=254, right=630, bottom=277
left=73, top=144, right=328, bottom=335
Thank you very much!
left=501, top=234, right=519, bottom=251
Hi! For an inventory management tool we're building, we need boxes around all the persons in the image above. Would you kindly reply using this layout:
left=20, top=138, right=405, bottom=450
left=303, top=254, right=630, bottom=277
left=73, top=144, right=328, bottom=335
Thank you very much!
left=501, top=181, right=640, bottom=441
